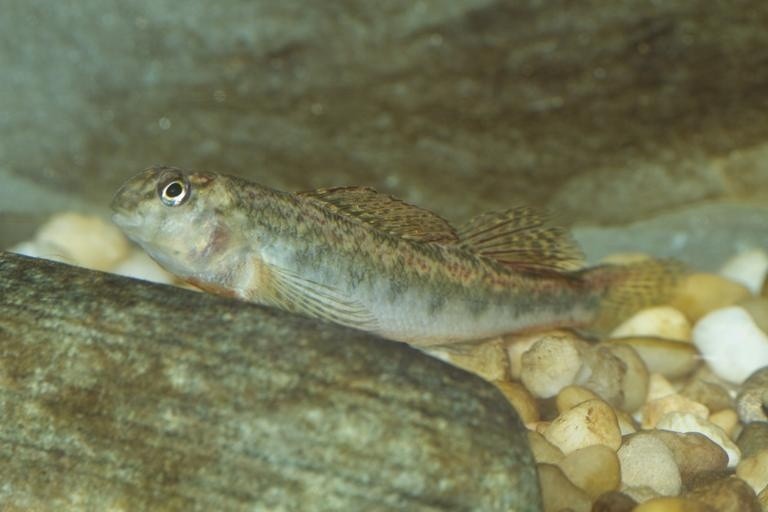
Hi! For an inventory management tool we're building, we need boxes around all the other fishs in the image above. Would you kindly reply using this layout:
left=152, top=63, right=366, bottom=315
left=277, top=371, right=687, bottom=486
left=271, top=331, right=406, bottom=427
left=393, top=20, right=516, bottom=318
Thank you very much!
left=108, top=161, right=690, bottom=349
